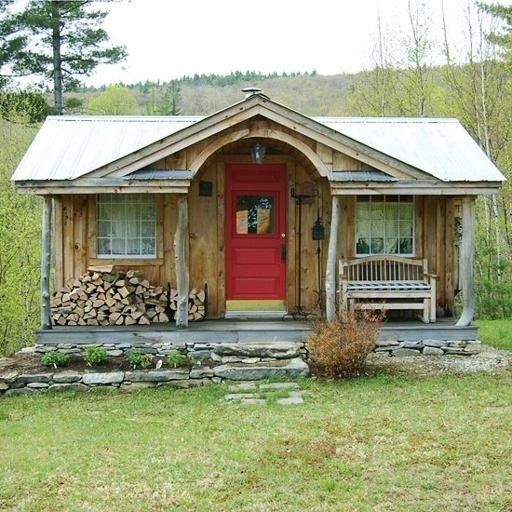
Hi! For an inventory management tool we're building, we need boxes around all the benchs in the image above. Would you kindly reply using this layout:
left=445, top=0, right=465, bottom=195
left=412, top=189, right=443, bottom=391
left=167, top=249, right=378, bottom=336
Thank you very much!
left=338, top=255, right=441, bottom=324
